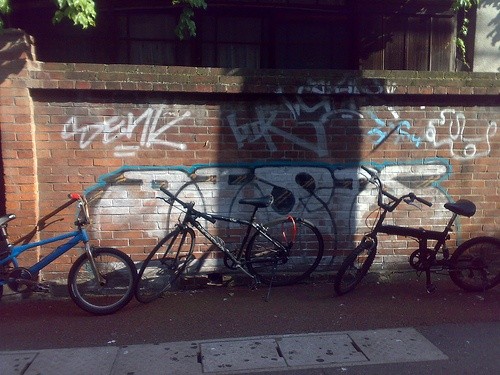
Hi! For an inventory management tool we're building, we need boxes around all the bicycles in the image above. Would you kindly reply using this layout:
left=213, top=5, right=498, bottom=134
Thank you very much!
left=0, top=192, right=138, bottom=315
left=132, top=187, right=326, bottom=305
left=334, top=165, right=500, bottom=297
